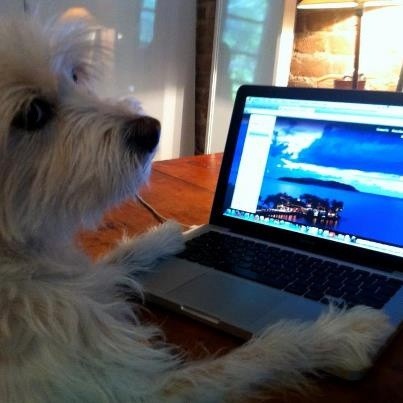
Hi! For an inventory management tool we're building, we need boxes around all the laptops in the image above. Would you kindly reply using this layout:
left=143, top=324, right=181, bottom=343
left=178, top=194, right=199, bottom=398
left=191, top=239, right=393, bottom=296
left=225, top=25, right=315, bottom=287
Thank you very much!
left=128, top=84, right=403, bottom=379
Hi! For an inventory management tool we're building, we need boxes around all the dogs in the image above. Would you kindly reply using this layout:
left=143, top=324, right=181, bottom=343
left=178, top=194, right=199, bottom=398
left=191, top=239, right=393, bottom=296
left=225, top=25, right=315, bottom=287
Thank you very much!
left=0, top=0, right=396, bottom=403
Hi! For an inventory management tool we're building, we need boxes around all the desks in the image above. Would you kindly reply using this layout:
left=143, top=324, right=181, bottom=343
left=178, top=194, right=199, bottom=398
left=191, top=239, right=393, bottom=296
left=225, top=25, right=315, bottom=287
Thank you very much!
left=73, top=149, right=403, bottom=403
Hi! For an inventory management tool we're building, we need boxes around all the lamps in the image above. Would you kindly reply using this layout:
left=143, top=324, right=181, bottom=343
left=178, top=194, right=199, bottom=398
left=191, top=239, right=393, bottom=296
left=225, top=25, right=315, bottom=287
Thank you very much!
left=297, top=0, right=400, bottom=93
